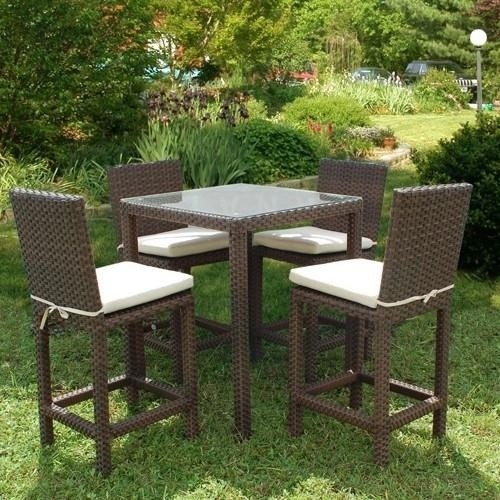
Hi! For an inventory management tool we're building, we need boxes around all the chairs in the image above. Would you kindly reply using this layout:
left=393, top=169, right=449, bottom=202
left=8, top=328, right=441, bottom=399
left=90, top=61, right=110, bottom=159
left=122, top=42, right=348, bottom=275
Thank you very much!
left=250, top=160, right=388, bottom=373
left=286, top=179, right=472, bottom=470
left=10, top=186, right=203, bottom=477
left=107, top=158, right=263, bottom=383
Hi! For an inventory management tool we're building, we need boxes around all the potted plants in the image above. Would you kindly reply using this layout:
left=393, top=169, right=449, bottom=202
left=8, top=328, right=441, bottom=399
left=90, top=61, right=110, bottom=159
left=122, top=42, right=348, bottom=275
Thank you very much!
left=373, top=125, right=396, bottom=154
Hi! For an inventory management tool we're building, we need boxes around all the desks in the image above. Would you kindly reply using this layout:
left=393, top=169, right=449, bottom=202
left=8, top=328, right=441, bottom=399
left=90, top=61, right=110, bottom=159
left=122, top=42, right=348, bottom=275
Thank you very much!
left=120, top=180, right=362, bottom=439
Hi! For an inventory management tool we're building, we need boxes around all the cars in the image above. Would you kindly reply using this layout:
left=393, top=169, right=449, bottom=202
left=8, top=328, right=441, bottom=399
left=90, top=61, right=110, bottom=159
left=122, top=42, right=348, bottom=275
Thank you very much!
left=90, top=34, right=210, bottom=86
left=348, top=60, right=473, bottom=104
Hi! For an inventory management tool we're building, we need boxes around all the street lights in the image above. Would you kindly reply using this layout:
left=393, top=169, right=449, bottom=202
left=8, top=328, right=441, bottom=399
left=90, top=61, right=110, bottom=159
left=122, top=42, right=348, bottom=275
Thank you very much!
left=470, top=28, right=488, bottom=109
